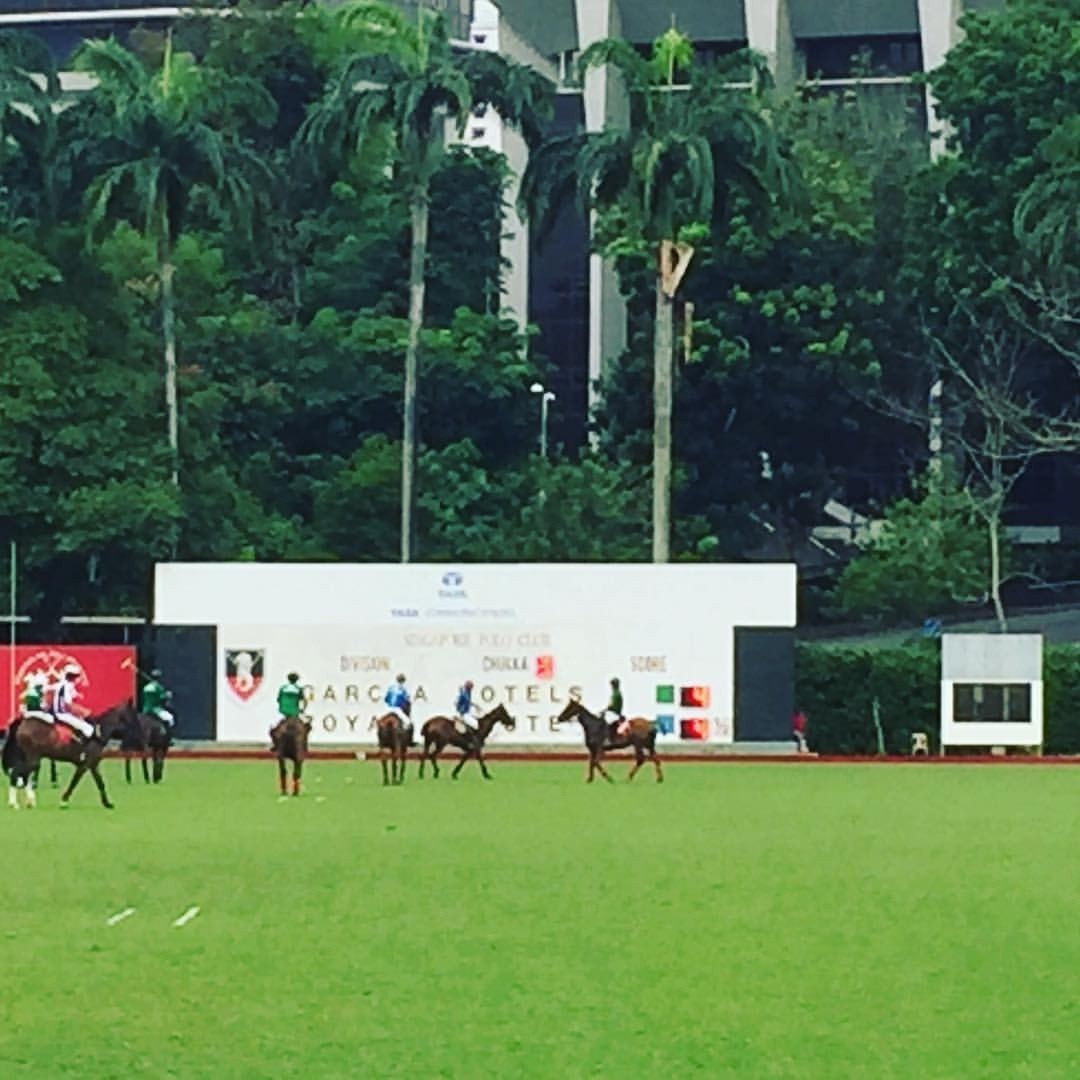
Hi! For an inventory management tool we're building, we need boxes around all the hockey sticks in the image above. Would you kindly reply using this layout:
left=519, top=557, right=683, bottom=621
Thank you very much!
left=119, top=657, right=173, bottom=698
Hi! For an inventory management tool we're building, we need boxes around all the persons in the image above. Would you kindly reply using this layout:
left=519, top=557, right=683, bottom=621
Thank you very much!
left=19, top=668, right=96, bottom=745
left=268, top=673, right=309, bottom=753
left=376, top=672, right=417, bottom=747
left=142, top=669, right=176, bottom=746
left=453, top=680, right=484, bottom=746
left=601, top=677, right=624, bottom=743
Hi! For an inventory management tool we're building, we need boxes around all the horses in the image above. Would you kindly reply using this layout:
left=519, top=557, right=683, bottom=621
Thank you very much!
left=1, top=696, right=142, bottom=810
left=419, top=703, right=515, bottom=780
left=123, top=712, right=172, bottom=784
left=272, top=716, right=311, bottom=798
left=559, top=698, right=664, bottom=784
left=377, top=715, right=408, bottom=786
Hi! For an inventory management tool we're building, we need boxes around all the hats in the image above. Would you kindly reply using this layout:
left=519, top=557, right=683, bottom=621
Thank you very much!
left=64, top=664, right=81, bottom=680
left=397, top=673, right=407, bottom=681
left=464, top=680, right=474, bottom=688
left=151, top=668, right=163, bottom=676
left=287, top=671, right=299, bottom=680
left=609, top=678, right=619, bottom=688
